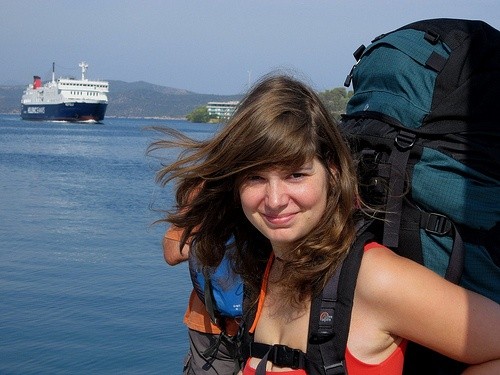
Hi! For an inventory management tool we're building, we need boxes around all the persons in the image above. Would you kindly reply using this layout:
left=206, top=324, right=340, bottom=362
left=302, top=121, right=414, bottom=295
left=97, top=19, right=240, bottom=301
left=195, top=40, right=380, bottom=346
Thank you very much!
left=163, top=169, right=248, bottom=375
left=144, top=72, right=500, bottom=375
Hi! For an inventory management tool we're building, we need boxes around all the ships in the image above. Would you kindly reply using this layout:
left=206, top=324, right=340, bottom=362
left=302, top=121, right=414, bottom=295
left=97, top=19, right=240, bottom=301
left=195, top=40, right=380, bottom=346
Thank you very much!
left=21, top=62, right=109, bottom=122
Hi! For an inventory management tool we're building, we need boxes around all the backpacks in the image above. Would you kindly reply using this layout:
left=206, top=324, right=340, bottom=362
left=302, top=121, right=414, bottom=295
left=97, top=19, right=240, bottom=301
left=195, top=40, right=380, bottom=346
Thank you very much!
left=191, top=208, right=244, bottom=348
left=238, top=19, right=500, bottom=375
